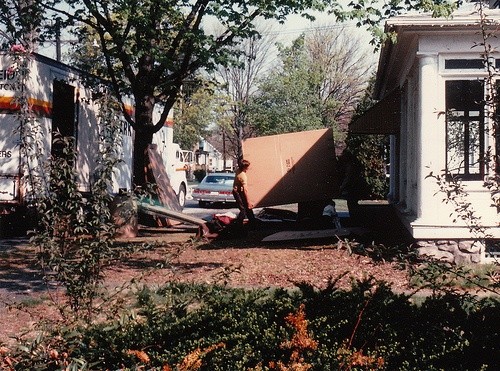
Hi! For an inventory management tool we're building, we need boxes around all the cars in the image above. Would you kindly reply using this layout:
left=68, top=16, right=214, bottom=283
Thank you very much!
left=191, top=173, right=235, bottom=207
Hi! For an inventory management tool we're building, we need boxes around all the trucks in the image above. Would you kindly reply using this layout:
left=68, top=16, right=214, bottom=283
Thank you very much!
left=0, top=49, right=189, bottom=211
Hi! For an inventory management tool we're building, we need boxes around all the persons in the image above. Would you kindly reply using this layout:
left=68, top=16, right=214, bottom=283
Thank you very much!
left=231, top=159, right=262, bottom=225
left=193, top=163, right=212, bottom=206
left=322, top=201, right=337, bottom=229
